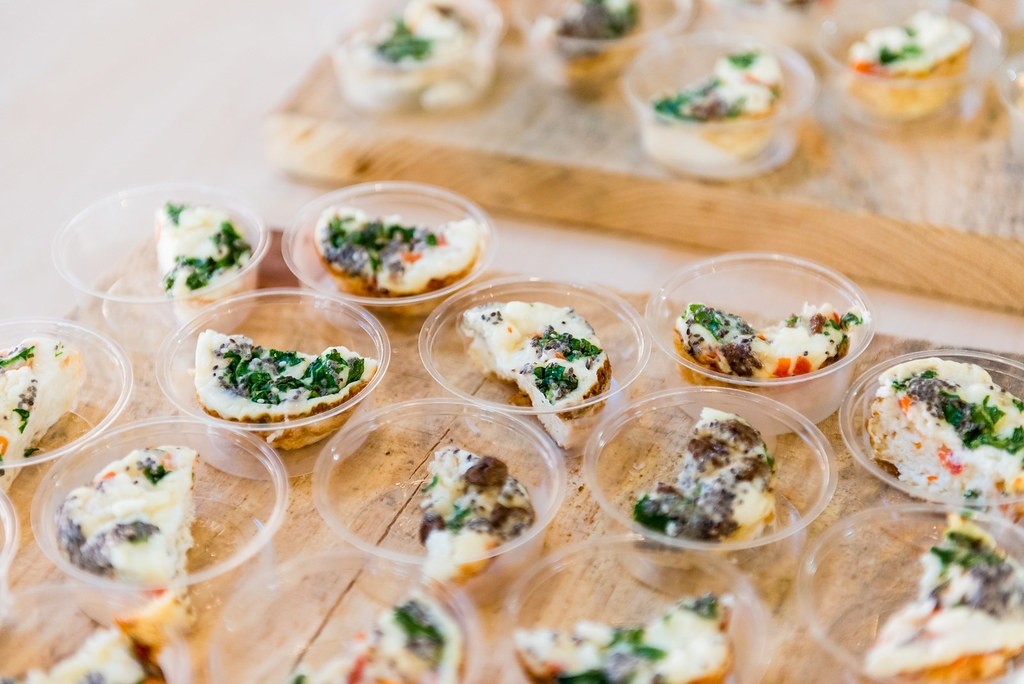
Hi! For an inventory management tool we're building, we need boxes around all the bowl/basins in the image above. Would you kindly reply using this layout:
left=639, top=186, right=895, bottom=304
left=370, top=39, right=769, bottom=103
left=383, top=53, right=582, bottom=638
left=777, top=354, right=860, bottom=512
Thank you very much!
left=30, top=417, right=290, bottom=636
left=1, top=490, right=22, bottom=582
left=309, top=399, right=566, bottom=600
left=645, top=253, right=875, bottom=436
left=515, top=1, right=691, bottom=98
left=623, top=35, right=813, bottom=179
left=1001, top=50, right=1024, bottom=160
left=817, top=0, right=1004, bottom=137
left=794, top=503, right=1024, bottom=683
left=584, top=385, right=836, bottom=554
left=1, top=321, right=132, bottom=549
left=838, top=348, right=1024, bottom=530
left=500, top=528, right=771, bottom=683
left=1, top=585, right=197, bottom=684
left=279, top=184, right=505, bottom=330
left=162, top=289, right=390, bottom=478
left=208, top=550, right=487, bottom=684
left=331, top=1, right=504, bottom=109
left=56, top=182, right=273, bottom=353
left=419, top=274, right=652, bottom=443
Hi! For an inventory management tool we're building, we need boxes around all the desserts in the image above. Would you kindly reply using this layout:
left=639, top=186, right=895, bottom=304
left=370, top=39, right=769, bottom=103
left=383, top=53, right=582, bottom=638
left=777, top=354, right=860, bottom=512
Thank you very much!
left=0, top=0, right=1024, bottom=684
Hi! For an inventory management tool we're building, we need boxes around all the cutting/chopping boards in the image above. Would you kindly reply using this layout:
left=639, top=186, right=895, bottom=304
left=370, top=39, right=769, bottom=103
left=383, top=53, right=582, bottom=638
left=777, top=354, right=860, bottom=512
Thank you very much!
left=1, top=227, right=1021, bottom=684
left=265, top=4, right=1022, bottom=316
